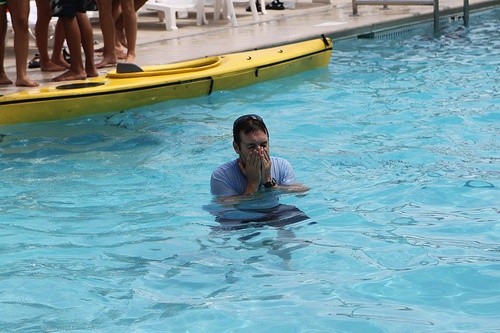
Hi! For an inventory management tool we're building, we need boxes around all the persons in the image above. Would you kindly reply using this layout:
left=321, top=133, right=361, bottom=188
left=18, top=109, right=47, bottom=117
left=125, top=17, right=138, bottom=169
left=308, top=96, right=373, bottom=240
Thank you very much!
left=210, top=114, right=295, bottom=195
left=0, top=0, right=148, bottom=87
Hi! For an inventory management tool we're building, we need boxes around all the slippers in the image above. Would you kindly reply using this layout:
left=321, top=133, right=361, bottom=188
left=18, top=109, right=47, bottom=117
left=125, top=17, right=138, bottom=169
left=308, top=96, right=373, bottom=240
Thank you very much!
left=29, top=52, right=40, bottom=68
left=63, top=49, right=71, bottom=64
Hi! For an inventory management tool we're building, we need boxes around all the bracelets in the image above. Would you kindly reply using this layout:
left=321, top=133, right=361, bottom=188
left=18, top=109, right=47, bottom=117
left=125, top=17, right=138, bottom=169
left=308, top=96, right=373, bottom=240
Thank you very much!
left=264, top=178, right=277, bottom=188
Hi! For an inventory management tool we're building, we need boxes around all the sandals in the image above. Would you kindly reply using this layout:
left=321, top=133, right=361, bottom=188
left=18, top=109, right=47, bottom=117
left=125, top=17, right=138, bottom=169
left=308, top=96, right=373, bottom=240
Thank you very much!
left=246, top=0, right=261, bottom=11
left=266, top=0, right=285, bottom=10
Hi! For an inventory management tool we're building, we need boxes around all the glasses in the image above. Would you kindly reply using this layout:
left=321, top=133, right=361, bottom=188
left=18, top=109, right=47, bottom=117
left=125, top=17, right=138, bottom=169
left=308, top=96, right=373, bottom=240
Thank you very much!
left=234, top=115, right=263, bottom=127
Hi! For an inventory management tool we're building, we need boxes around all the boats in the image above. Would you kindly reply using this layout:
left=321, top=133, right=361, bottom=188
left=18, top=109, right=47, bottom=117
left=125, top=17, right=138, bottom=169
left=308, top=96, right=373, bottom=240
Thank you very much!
left=0, top=33, right=333, bottom=125
